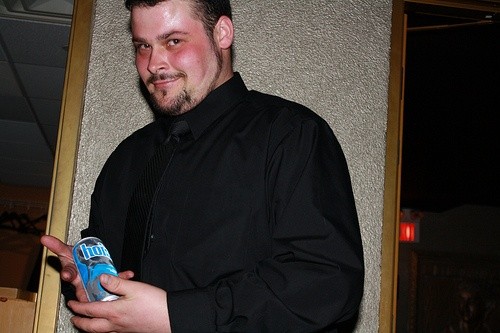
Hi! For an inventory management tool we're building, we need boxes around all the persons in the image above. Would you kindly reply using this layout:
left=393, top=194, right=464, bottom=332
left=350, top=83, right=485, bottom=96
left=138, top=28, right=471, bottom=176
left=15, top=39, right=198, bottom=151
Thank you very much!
left=40, top=1, right=366, bottom=333
